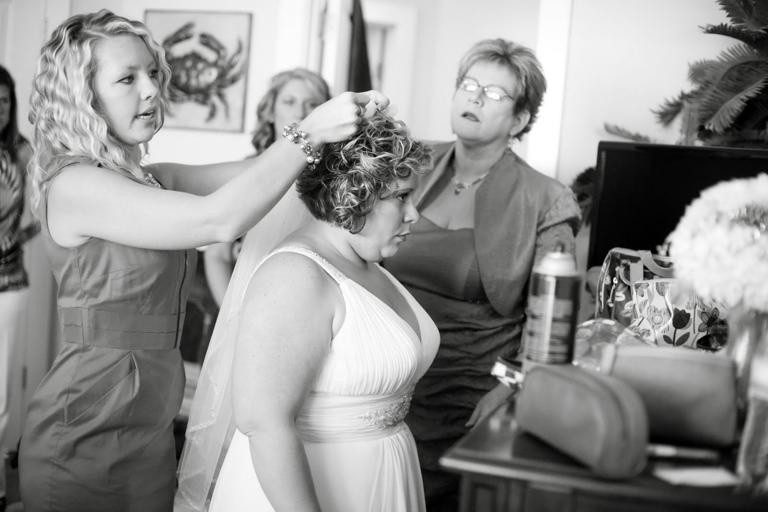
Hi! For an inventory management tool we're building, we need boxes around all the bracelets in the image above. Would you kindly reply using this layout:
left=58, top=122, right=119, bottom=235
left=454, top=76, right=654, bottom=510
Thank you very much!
left=283, top=122, right=321, bottom=169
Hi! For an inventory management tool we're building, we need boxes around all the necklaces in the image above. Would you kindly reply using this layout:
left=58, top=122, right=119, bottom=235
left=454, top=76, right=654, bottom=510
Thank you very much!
left=445, top=164, right=488, bottom=196
left=107, top=160, right=161, bottom=187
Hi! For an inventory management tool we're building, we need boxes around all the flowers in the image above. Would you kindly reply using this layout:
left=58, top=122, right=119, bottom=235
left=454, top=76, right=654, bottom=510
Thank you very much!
left=667, top=172, right=768, bottom=334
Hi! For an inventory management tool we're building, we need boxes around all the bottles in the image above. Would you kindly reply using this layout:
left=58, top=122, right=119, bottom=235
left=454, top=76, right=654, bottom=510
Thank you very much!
left=520, top=242, right=582, bottom=367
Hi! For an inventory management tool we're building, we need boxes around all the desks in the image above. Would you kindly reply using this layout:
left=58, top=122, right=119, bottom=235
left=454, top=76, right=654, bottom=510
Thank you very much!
left=435, top=401, right=766, bottom=512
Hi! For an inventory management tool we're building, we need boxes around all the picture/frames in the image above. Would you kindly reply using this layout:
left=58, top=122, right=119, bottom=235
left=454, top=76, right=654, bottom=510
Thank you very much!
left=143, top=10, right=251, bottom=133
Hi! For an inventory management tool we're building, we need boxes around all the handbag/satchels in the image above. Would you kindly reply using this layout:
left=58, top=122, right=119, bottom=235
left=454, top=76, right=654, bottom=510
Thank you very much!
left=516, top=363, right=651, bottom=479
left=593, top=245, right=673, bottom=327
left=599, top=343, right=739, bottom=447
left=614, top=277, right=730, bottom=354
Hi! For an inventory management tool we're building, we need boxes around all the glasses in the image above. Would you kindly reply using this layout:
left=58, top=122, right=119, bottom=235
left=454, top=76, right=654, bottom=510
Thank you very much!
left=455, top=76, right=516, bottom=103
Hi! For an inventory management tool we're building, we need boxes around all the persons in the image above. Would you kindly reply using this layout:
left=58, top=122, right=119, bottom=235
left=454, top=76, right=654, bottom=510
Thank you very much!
left=203, top=65, right=333, bottom=310
left=16, top=8, right=392, bottom=512
left=379, top=37, right=584, bottom=512
left=0, top=66, right=40, bottom=511
left=169, top=106, right=444, bottom=512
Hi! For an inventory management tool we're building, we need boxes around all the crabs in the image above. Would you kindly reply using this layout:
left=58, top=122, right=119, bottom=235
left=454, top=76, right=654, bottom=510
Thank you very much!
left=158, top=21, right=246, bottom=123
